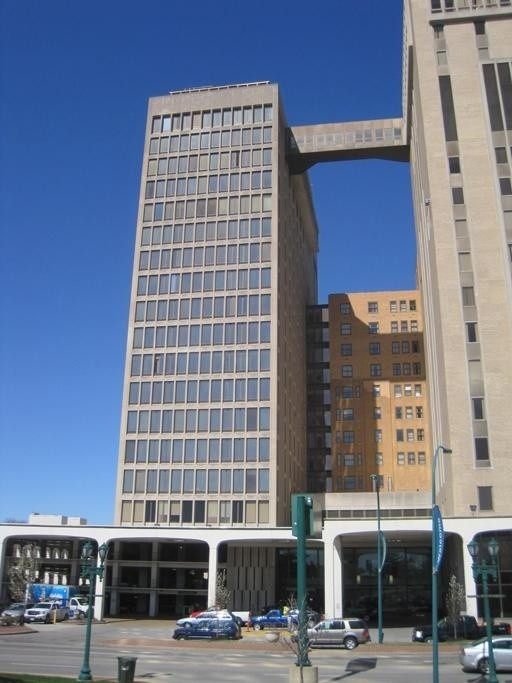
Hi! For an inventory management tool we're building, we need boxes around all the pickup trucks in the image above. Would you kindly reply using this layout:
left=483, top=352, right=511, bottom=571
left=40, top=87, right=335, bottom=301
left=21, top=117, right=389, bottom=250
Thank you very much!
left=412, top=613, right=512, bottom=645
left=24, top=601, right=71, bottom=623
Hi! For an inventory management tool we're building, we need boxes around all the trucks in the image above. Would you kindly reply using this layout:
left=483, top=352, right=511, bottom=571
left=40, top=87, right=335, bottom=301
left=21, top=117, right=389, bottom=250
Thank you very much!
left=26, top=583, right=94, bottom=619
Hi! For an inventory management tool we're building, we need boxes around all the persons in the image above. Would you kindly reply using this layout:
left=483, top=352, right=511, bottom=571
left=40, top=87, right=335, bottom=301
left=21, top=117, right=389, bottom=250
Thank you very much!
left=246, top=609, right=255, bottom=632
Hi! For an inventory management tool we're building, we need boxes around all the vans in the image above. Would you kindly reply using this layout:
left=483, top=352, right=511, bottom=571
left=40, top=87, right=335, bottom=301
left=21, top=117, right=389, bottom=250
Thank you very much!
left=2, top=600, right=36, bottom=624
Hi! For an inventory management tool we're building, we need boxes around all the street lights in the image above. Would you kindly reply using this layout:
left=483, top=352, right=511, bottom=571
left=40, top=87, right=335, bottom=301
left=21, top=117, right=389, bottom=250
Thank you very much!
left=371, top=471, right=389, bottom=642
left=76, top=540, right=110, bottom=681
left=428, top=442, right=453, bottom=681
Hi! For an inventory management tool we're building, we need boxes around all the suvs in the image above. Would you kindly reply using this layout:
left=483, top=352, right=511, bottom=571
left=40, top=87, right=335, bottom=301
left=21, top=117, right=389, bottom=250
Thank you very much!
left=173, top=606, right=371, bottom=649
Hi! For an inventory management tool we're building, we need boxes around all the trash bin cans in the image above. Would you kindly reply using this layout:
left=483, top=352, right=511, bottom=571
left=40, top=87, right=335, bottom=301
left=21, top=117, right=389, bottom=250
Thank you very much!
left=117, top=656, right=137, bottom=683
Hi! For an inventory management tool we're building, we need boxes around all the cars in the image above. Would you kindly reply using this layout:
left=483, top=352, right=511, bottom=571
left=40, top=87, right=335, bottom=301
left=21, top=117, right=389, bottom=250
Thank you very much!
left=459, top=634, right=512, bottom=673
